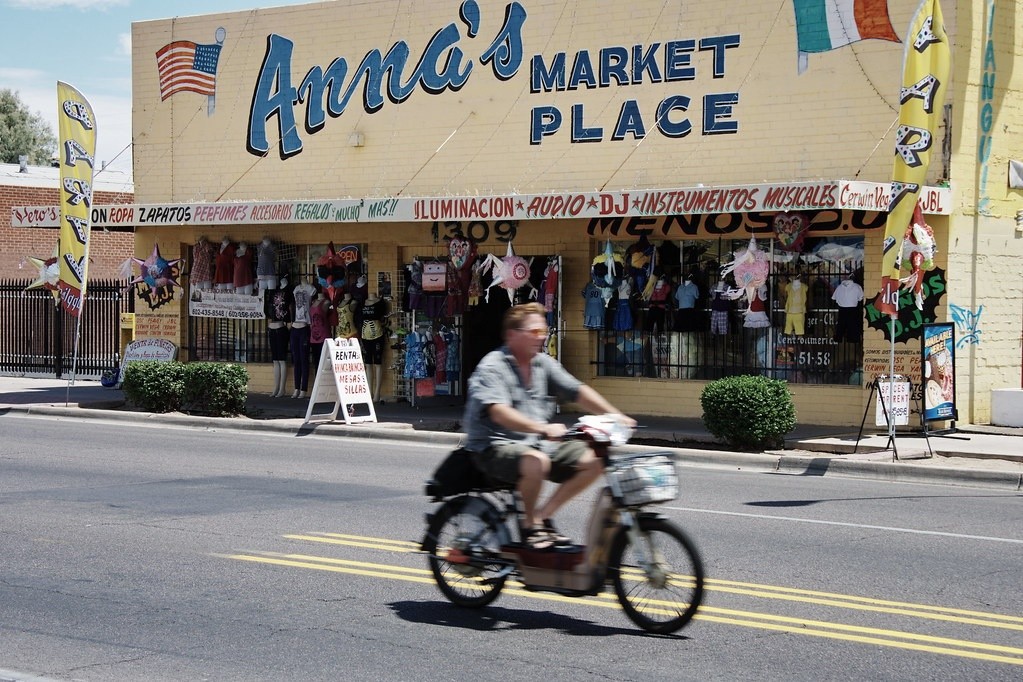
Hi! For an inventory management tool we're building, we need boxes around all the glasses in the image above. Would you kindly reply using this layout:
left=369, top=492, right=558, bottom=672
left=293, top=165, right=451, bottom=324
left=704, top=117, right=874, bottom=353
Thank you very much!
left=513, top=328, right=550, bottom=336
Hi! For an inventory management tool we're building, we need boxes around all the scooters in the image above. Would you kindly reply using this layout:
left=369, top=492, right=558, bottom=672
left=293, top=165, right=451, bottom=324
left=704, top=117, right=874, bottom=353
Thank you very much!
left=412, top=419, right=708, bottom=638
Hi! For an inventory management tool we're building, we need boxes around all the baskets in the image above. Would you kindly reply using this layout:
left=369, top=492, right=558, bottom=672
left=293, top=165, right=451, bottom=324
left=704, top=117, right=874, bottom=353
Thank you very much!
left=606, top=451, right=679, bottom=508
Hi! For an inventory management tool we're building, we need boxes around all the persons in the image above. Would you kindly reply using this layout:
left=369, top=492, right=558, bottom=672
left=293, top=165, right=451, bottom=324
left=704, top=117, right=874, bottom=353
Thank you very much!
left=831, top=280, right=865, bottom=341
left=423, top=330, right=436, bottom=376
left=267, top=322, right=289, bottom=397
left=592, top=242, right=624, bottom=285
left=581, top=279, right=607, bottom=329
left=675, top=281, right=700, bottom=333
left=213, top=236, right=235, bottom=290
left=256, top=238, right=278, bottom=289
left=743, top=283, right=773, bottom=327
left=188, top=235, right=213, bottom=288
left=627, top=239, right=657, bottom=294
left=614, top=280, right=635, bottom=329
left=459, top=302, right=638, bottom=549
left=361, top=293, right=391, bottom=402
left=289, top=322, right=311, bottom=399
left=335, top=294, right=359, bottom=340
left=271, top=276, right=295, bottom=323
left=785, top=278, right=808, bottom=335
left=232, top=241, right=254, bottom=295
left=307, top=290, right=333, bottom=376
left=294, top=275, right=318, bottom=324
left=434, top=331, right=448, bottom=384
left=648, top=278, right=672, bottom=334
left=444, top=331, right=461, bottom=381
left=544, top=260, right=559, bottom=312
left=405, top=333, right=427, bottom=378
left=710, top=281, right=733, bottom=334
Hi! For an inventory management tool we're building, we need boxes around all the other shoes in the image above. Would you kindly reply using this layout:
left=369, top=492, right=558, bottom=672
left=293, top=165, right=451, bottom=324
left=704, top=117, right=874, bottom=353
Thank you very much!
left=523, top=525, right=573, bottom=549
